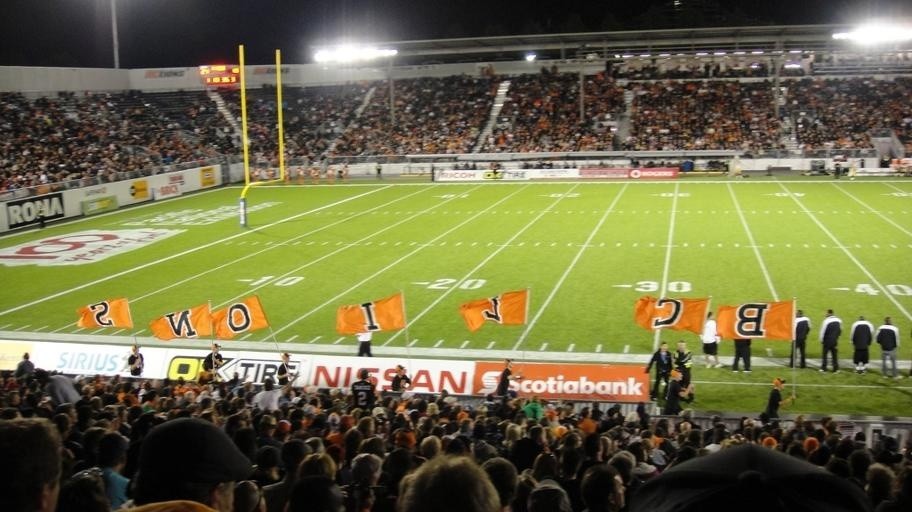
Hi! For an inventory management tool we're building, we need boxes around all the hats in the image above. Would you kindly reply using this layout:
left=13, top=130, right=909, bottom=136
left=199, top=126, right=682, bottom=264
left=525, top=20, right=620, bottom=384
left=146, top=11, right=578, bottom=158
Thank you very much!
left=142, top=417, right=258, bottom=483
left=258, top=402, right=596, bottom=448
left=627, top=437, right=873, bottom=512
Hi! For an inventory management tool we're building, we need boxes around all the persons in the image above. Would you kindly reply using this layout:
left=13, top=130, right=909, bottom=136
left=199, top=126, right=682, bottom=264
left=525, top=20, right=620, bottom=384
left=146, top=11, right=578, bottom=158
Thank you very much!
left=872, top=315, right=904, bottom=380
left=37, top=204, right=46, bottom=230
left=848, top=314, right=874, bottom=376
left=788, top=310, right=811, bottom=370
left=697, top=310, right=723, bottom=368
left=817, top=308, right=843, bottom=375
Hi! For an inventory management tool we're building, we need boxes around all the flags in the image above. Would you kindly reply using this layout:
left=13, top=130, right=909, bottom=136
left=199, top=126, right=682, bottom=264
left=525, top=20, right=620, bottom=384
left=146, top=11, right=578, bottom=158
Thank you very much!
left=75, top=297, right=135, bottom=329
left=631, top=295, right=711, bottom=335
left=459, top=287, right=532, bottom=331
left=332, top=291, right=409, bottom=337
left=209, top=294, right=268, bottom=340
left=146, top=303, right=213, bottom=342
left=715, top=297, right=798, bottom=340
